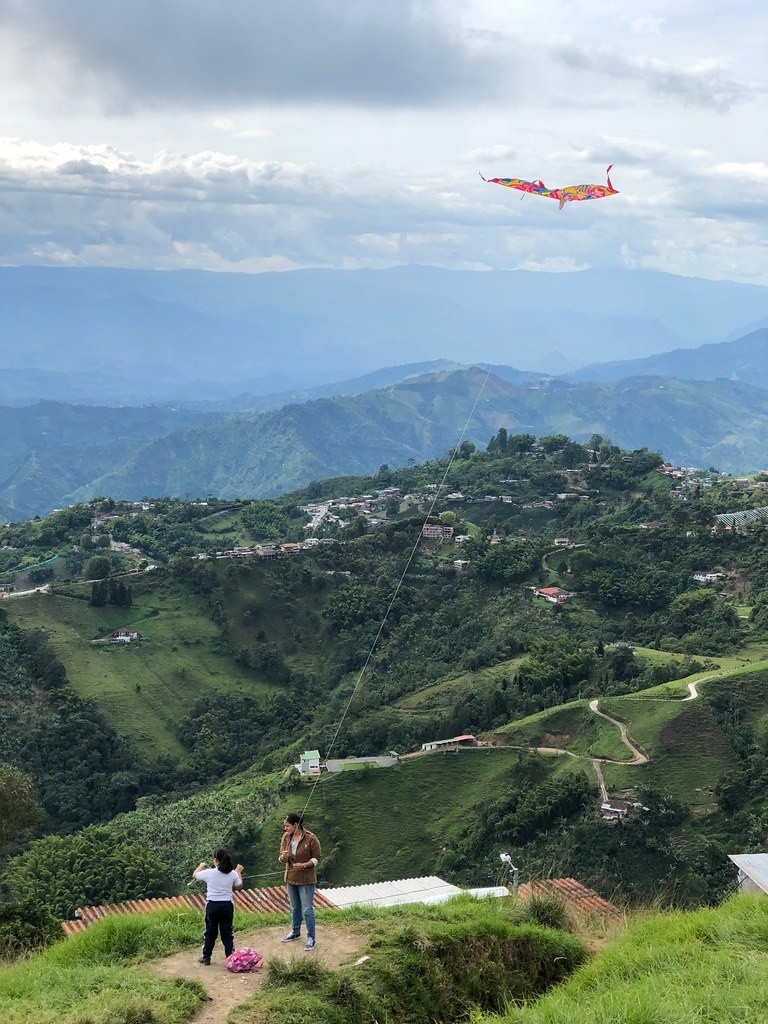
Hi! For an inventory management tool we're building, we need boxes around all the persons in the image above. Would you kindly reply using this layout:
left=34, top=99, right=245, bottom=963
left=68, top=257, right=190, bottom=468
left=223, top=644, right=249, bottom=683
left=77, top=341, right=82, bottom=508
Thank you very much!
left=278, top=812, right=320, bottom=950
left=193, top=848, right=244, bottom=966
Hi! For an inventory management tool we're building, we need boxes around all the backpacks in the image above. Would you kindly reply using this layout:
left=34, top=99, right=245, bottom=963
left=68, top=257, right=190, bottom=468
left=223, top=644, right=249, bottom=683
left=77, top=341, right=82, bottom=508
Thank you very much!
left=226, top=947, right=263, bottom=973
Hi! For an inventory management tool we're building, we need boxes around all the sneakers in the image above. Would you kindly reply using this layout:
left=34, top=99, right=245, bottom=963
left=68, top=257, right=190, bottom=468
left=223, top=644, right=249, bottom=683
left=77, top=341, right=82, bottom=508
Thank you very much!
left=304, top=937, right=316, bottom=951
left=281, top=931, right=301, bottom=942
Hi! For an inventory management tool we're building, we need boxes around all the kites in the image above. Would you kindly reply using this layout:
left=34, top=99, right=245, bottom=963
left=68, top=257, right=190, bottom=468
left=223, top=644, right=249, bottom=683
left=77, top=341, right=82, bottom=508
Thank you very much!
left=479, top=163, right=622, bottom=210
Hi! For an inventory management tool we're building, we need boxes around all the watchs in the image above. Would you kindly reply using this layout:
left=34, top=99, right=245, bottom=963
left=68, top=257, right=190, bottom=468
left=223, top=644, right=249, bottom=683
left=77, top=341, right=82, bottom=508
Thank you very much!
left=303, top=863, right=306, bottom=869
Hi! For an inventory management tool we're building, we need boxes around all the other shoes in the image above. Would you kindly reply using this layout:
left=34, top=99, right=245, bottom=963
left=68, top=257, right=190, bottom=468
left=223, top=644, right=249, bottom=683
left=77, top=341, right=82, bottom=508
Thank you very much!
left=198, top=957, right=210, bottom=965
